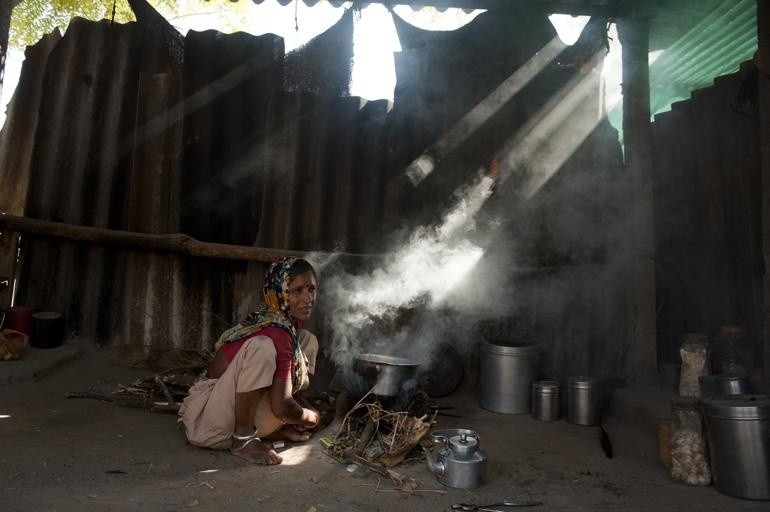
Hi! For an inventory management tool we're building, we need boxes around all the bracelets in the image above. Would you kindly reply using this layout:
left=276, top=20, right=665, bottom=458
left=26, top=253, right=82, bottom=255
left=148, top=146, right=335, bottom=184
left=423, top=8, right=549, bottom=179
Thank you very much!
left=303, top=413, right=320, bottom=429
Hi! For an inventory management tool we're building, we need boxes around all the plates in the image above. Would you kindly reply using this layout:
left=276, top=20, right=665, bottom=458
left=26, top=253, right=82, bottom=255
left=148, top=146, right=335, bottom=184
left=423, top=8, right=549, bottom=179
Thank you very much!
left=484, top=336, right=542, bottom=347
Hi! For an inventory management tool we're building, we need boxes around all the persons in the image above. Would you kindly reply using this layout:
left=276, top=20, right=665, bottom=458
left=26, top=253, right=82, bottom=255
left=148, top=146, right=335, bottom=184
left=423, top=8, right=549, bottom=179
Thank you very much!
left=175, top=254, right=322, bottom=468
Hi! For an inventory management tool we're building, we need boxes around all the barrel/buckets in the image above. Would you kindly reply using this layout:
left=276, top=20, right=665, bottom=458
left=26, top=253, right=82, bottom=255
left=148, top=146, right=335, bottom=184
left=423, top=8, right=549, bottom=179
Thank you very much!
left=6, top=306, right=33, bottom=336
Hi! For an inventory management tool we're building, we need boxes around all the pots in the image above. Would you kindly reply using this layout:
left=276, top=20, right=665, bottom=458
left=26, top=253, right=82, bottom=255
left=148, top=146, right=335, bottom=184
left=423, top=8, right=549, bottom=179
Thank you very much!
left=350, top=352, right=421, bottom=399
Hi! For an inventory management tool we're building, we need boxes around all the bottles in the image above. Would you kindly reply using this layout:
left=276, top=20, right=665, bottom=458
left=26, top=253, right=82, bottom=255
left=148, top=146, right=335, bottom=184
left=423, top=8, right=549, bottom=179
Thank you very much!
left=711, top=324, right=757, bottom=379
left=669, top=394, right=711, bottom=485
left=678, top=333, right=711, bottom=399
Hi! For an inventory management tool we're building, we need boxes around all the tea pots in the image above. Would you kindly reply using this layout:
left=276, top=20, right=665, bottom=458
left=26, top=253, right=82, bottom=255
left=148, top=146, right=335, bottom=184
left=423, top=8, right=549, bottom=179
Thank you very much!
left=421, top=428, right=488, bottom=489
